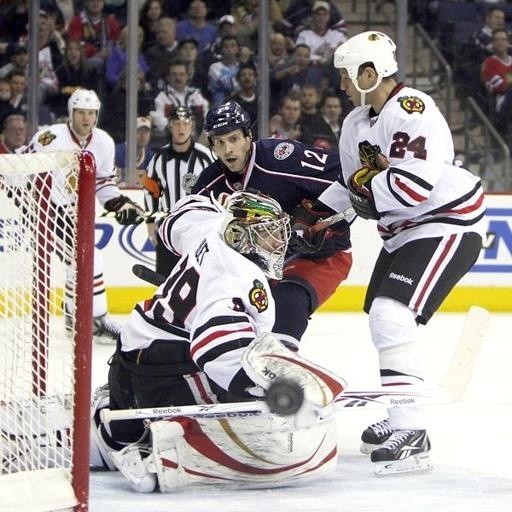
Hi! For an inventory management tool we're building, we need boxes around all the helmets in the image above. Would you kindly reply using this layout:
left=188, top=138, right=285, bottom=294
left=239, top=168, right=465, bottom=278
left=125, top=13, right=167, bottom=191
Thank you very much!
left=333, top=31, right=399, bottom=78
left=168, top=99, right=251, bottom=146
left=223, top=192, right=290, bottom=250
left=67, top=88, right=102, bottom=124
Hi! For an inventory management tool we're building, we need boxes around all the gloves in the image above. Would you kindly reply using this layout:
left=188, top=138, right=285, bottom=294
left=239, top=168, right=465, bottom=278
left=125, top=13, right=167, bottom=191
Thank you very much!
left=112, top=194, right=145, bottom=224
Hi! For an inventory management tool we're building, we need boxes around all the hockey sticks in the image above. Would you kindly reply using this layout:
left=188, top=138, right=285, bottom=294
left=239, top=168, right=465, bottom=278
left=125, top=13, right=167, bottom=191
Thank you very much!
left=100, top=304, right=490, bottom=424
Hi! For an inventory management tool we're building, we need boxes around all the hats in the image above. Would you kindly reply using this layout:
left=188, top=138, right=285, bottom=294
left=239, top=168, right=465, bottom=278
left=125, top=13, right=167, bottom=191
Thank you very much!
left=136, top=116, right=151, bottom=131
left=218, top=15, right=235, bottom=25
left=311, top=0, right=331, bottom=12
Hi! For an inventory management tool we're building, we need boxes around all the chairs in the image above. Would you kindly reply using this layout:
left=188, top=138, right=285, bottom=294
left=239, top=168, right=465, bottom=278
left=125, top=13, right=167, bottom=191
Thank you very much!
left=408, top=0, right=512, bottom=65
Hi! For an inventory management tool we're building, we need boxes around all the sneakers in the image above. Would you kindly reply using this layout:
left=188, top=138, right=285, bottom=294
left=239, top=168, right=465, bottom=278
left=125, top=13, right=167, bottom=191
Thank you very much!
left=361, top=417, right=431, bottom=461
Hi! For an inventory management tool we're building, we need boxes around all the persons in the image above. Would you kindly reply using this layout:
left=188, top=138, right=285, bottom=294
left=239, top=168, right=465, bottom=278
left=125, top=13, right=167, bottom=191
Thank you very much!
left=1, top=0, right=354, bottom=495
left=290, top=29, right=489, bottom=476
left=407, top=0, right=512, bottom=162
